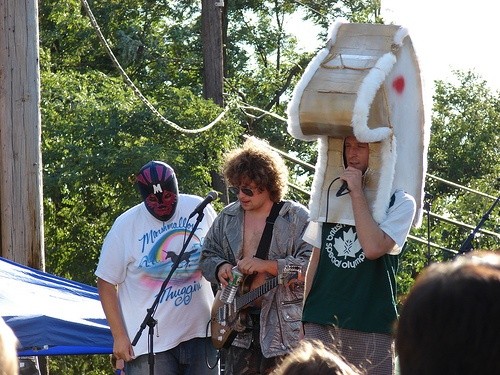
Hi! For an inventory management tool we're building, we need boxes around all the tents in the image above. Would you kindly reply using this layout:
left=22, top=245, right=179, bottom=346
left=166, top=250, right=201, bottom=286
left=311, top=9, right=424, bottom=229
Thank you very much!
left=0, top=257, right=114, bottom=375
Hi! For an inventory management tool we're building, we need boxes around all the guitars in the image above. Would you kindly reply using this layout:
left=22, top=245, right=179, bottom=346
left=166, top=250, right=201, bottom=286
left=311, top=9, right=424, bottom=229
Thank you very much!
left=211, top=264, right=302, bottom=349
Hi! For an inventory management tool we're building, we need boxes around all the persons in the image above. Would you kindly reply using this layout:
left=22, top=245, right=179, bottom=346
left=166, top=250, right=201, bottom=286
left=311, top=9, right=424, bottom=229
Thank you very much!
left=301, top=136, right=416, bottom=375
left=394, top=249, right=500, bottom=375
left=199, top=138, right=313, bottom=375
left=275, top=339, right=358, bottom=375
left=0, top=317, right=19, bottom=375
left=93, top=159, right=218, bottom=375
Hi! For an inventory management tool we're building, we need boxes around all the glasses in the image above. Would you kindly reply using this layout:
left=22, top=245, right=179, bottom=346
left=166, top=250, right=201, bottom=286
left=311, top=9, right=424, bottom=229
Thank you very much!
left=227, top=185, right=260, bottom=197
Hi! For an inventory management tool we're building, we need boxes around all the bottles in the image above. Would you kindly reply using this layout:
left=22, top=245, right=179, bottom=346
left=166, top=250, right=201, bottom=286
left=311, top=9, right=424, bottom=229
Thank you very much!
left=219, top=260, right=243, bottom=304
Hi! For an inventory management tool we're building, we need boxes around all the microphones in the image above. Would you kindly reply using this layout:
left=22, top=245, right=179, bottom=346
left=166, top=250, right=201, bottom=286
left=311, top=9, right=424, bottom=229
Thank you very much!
left=188, top=190, right=219, bottom=218
left=424, top=190, right=434, bottom=199
left=335, top=180, right=348, bottom=197
